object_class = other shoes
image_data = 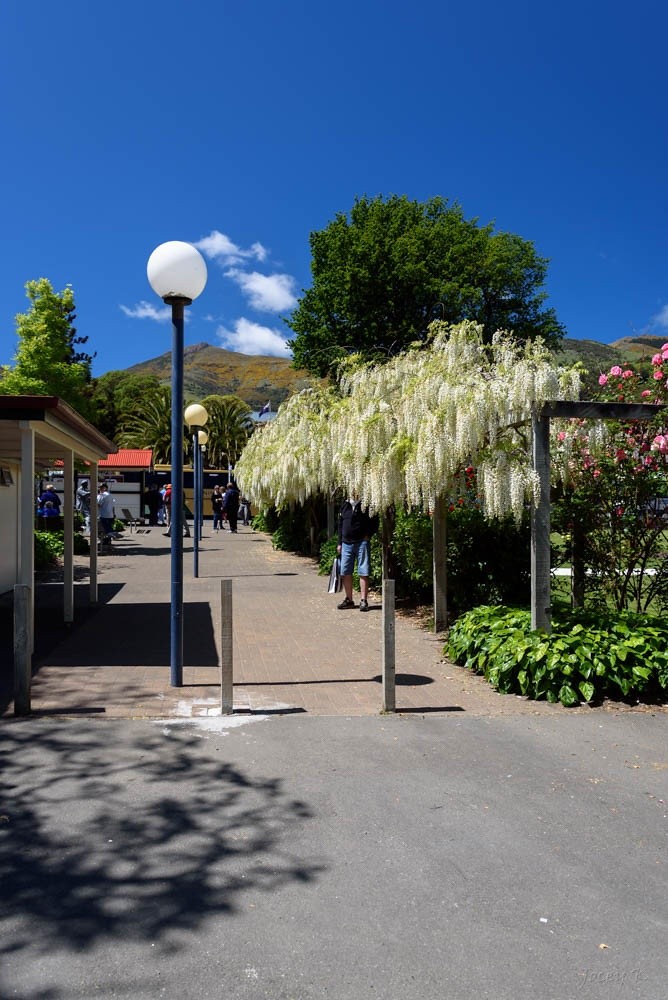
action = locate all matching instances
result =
[228,530,234,533]
[162,533,170,537]
[183,534,190,537]
[213,528,219,530]
[234,529,237,533]
[220,527,225,529]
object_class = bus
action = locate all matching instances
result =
[153,463,259,519]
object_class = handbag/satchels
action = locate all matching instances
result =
[327,555,343,594]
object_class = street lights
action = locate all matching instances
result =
[146,240,207,688]
[193,430,208,541]
[184,404,209,577]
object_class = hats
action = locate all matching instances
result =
[166,484,172,488]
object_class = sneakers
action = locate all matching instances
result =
[338,597,354,609]
[359,599,369,611]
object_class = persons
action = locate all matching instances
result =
[211,483,251,533]
[38,485,61,531]
[78,480,90,534]
[337,489,380,611]
[98,484,114,545]
[144,484,190,537]
